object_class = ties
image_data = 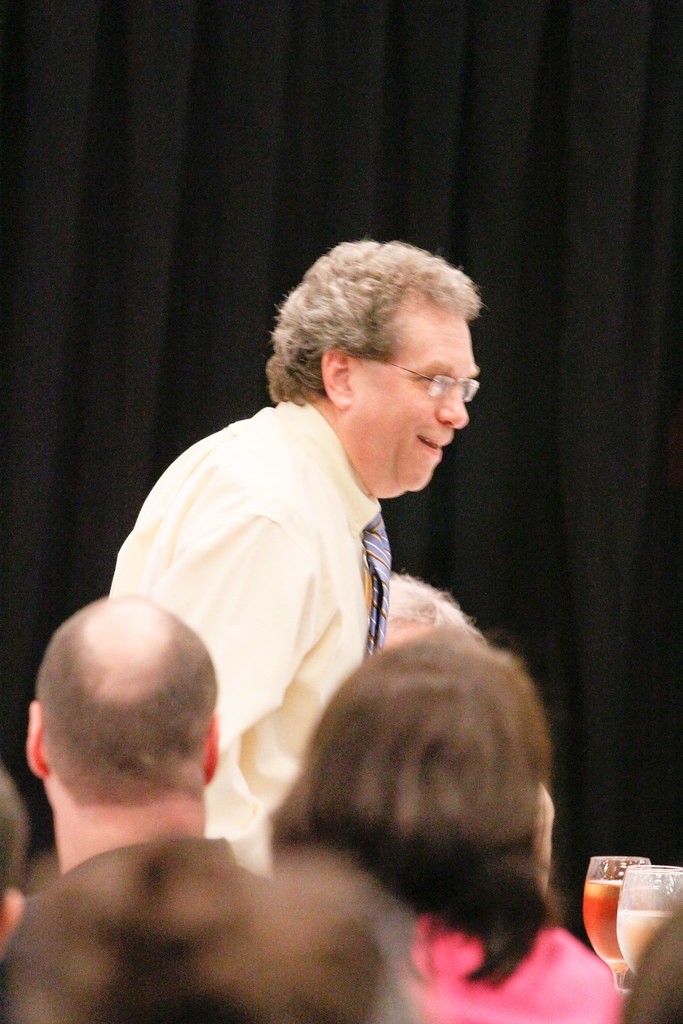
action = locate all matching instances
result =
[361,512,390,660]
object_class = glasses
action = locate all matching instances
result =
[367,352,480,402]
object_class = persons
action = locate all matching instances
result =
[0,575,683,1024]
[109,237,481,874]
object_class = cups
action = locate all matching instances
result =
[615,865,683,977]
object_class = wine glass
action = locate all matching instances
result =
[583,855,653,994]
